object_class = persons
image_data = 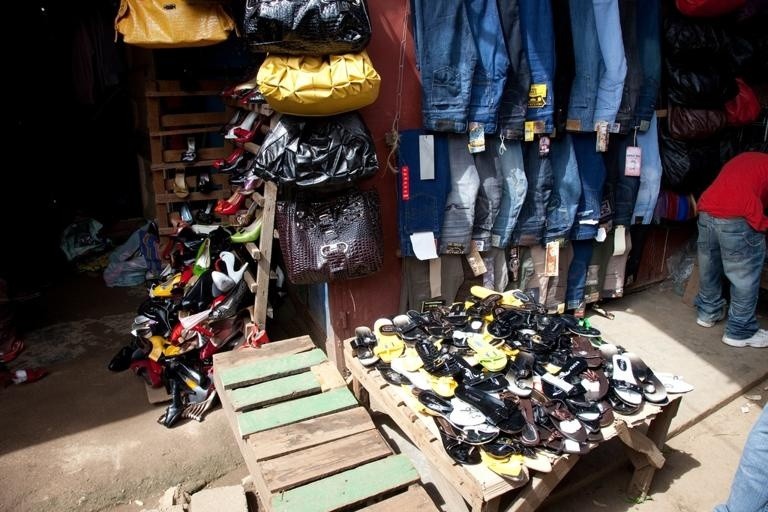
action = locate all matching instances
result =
[695,151,768,348]
[714,401,768,512]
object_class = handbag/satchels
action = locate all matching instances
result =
[273,190,388,286]
[240,0,376,57]
[250,110,383,193]
[254,48,383,120]
[112,0,243,52]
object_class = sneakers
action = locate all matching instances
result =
[720,328,768,348]
[695,303,726,328]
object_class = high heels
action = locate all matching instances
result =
[212,82,268,197]
[197,165,212,195]
[108,297,269,428]
[173,168,189,199]
[149,187,264,297]
[182,136,197,163]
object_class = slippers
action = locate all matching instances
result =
[422,286,556,482]
[615,345,693,416]
[350,309,421,383]
[558,313,615,456]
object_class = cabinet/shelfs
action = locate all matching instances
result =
[131,76,235,238]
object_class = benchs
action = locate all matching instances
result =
[209,333,440,510]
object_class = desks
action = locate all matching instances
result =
[341,289,681,511]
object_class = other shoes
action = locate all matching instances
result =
[178,70,200,95]
